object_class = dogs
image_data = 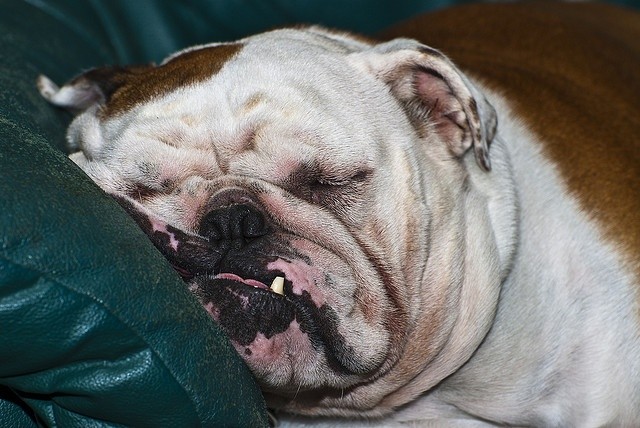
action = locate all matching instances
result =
[37,25,639,428]
